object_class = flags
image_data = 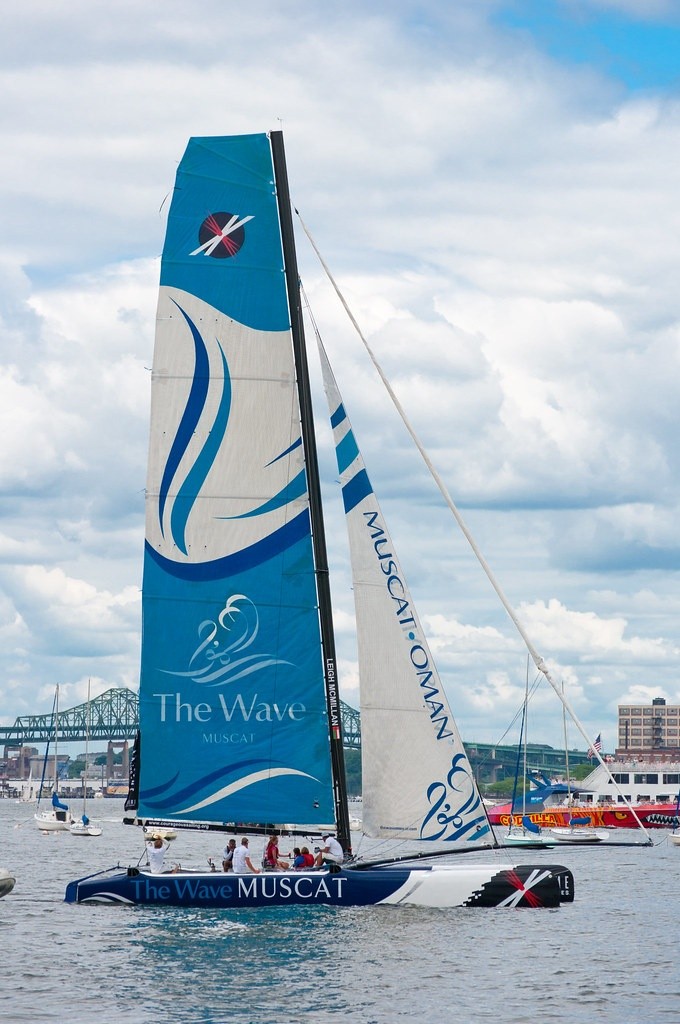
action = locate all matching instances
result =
[588,734,602,758]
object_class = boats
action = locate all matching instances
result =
[316,814,362,831]
[486,732,680,829]
[668,833,680,846]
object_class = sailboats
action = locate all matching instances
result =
[33,681,76,830]
[37,756,129,799]
[549,681,611,842]
[503,653,559,850]
[19,767,37,803]
[65,130,575,912]
[69,678,104,836]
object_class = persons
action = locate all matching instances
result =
[313,831,347,863]
[233,838,260,874]
[145,836,179,874]
[299,847,314,867]
[222,838,236,871]
[290,848,304,868]
[264,836,290,867]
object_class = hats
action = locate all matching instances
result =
[321,832,329,838]
